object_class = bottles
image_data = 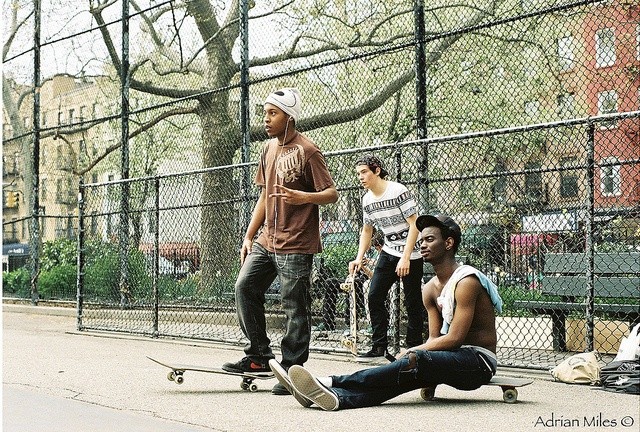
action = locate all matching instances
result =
[265,87,303,126]
[353,154,389,179]
[415,213,461,243]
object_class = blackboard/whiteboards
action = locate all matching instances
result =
[128,241,201,280]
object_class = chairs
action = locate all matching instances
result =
[221,356,275,377]
[287,364,341,412]
[365,345,387,357]
[271,381,293,394]
[268,358,315,408]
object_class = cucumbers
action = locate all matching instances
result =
[146,357,275,392]
[340,265,358,356]
[421,376,533,403]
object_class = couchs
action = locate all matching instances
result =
[5,192,19,208]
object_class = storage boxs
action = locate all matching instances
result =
[221,252,284,301]
[512,252,639,355]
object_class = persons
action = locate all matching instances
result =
[348,154,423,363]
[267,274,280,297]
[222,87,339,395]
[359,233,385,350]
[268,215,497,412]
[314,225,377,343]
[309,257,338,341]
